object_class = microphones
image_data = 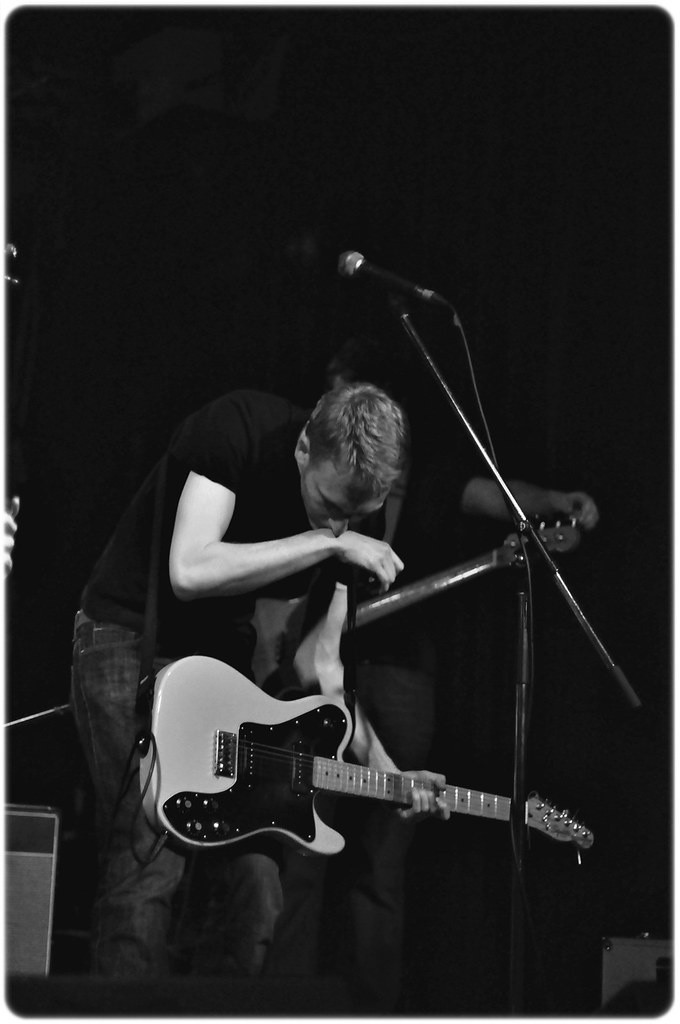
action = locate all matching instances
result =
[339,250,456,313]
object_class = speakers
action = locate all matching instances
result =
[6,804,61,976]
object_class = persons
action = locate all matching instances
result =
[59,379,406,986]
[4,492,25,582]
[268,285,600,1014]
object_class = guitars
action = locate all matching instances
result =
[133,655,596,868]
